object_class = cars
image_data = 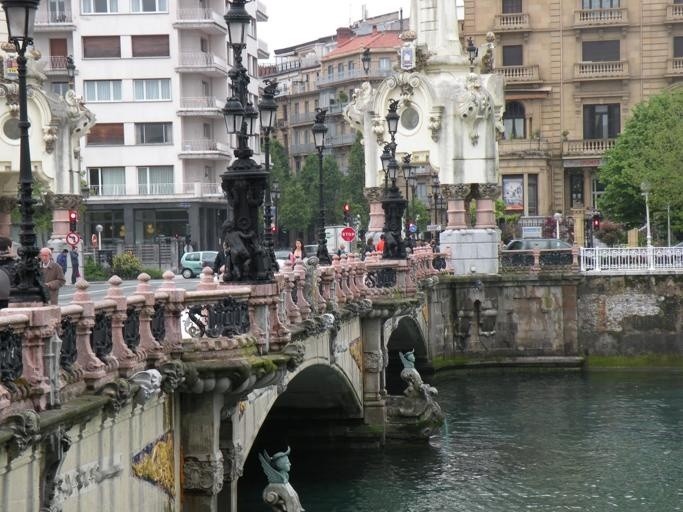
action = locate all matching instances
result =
[275,242,318,269]
[501,236,591,264]
[180,251,220,279]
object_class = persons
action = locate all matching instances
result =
[267,445,293,482]
[375,233,384,253]
[55,248,69,277]
[37,246,67,306]
[287,237,308,273]
[207,246,226,282]
[403,231,413,248]
[360,237,375,261]
[69,247,79,284]
[496,216,512,245]
[403,350,417,364]
[335,243,345,257]
[0,235,21,308]
[552,208,562,226]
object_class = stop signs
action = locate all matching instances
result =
[342,227,356,242]
[91,234,97,247]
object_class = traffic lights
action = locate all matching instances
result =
[67,208,79,231]
[343,202,351,222]
[594,213,599,229]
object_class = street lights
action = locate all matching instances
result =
[553,210,562,245]
[639,180,655,268]
[379,103,413,256]
[1,0,48,307]
[311,108,331,263]
[95,223,103,249]
[221,0,279,283]
[431,172,442,267]
